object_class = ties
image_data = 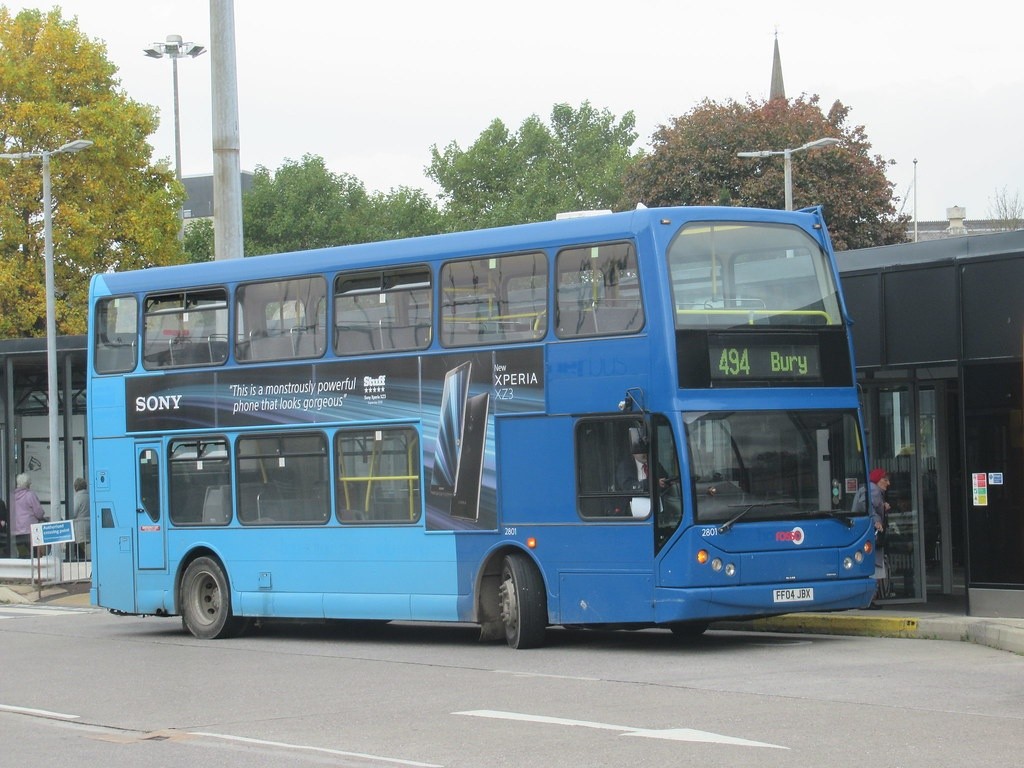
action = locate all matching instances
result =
[643,463,648,478]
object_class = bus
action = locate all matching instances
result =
[85,202,873,649]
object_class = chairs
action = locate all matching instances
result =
[131,298,771,366]
[254,489,408,521]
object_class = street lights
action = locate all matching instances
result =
[0,140,92,566]
[142,35,207,251]
[736,136,839,209]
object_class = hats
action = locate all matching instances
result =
[632,443,649,454]
[870,469,886,483]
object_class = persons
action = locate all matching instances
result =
[0,499,8,533]
[13,472,45,558]
[69,477,90,559]
[849,467,895,612]
[615,434,673,516]
